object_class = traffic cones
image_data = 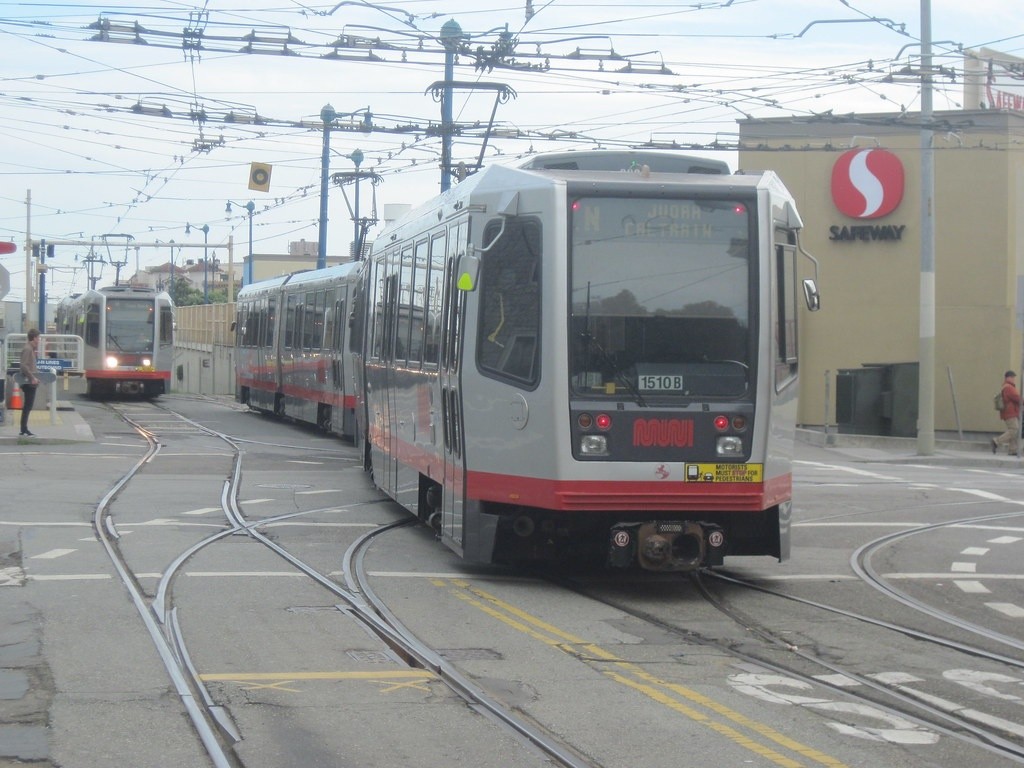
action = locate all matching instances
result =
[7,381,23,410]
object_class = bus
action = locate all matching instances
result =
[231,259,363,441]
[53,292,82,360]
[355,148,821,576]
[65,286,174,401]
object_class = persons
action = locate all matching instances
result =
[990,371,1021,456]
[17,330,40,435]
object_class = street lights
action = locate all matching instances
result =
[155,238,175,306]
[185,221,209,304]
[225,200,256,285]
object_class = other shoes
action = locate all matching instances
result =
[1009,453,1018,455]
[991,440,997,453]
[20,431,35,437]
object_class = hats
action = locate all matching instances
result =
[1005,372,1016,376]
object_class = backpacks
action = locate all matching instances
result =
[994,384,1012,410]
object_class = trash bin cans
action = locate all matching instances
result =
[836,368,885,436]
[863,362,921,438]
